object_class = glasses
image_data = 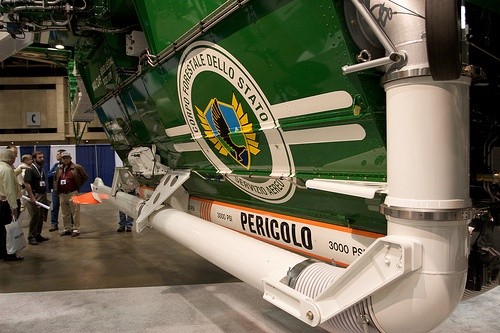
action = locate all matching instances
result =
[38,157,45,159]
[62,157,69,159]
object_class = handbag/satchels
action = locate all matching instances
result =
[42,198,51,222]
[5,214,29,255]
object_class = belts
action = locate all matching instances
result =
[62,189,76,194]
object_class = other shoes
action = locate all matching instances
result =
[4,254,24,261]
[59,231,71,236]
[126,227,132,232]
[72,232,80,237]
[37,236,49,241]
[48,226,58,231]
[117,227,125,232]
[28,239,39,245]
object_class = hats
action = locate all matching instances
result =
[61,151,72,159]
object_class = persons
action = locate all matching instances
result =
[0,145,49,261]
[54,152,88,237]
[117,189,138,232]
[47,149,74,231]
